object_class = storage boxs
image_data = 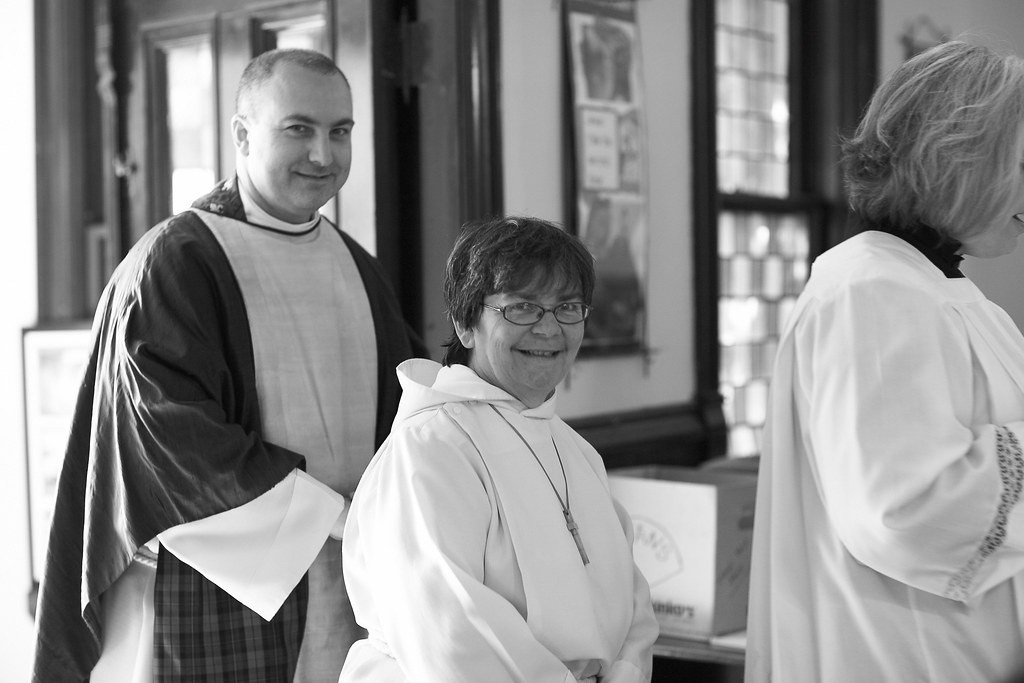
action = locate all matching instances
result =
[605,465,761,641]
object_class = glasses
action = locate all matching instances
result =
[484,302,593,326]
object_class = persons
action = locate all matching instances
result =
[338,215,660,683]
[32,49,435,683]
[744,35,1024,683]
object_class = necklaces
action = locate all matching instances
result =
[488,404,593,565]
